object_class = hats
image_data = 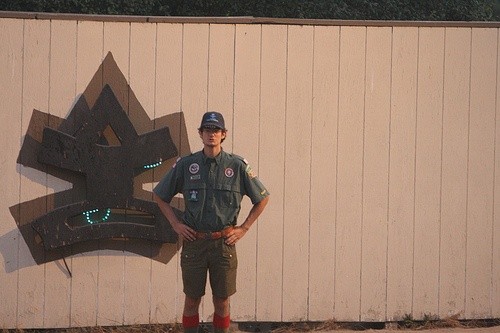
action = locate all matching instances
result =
[200,111,225,130]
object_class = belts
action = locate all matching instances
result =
[191,225,234,239]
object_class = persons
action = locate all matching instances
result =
[152,111,270,333]
